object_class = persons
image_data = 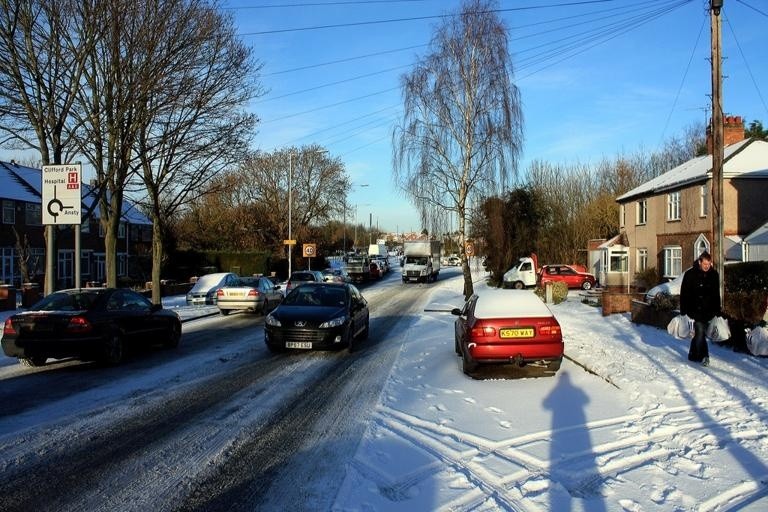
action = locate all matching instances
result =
[679,251,722,367]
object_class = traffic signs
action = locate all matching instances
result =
[303,243,316,257]
[464,241,476,256]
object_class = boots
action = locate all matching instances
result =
[703,357,709,366]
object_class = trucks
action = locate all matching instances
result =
[502,252,588,289]
[402,241,440,283]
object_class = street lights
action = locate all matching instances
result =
[343,183,370,255]
[289,150,330,279]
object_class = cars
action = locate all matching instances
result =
[451,290,563,370]
[442,257,462,266]
[1,287,182,367]
[644,259,743,309]
[540,263,595,290]
[186,257,390,351]
